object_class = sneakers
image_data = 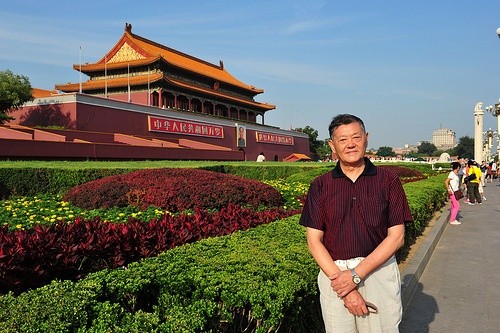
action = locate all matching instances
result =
[450,220,461,224]
[483,197,486,200]
[480,203,482,204]
[463,199,470,203]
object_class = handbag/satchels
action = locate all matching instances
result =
[464,174,476,183]
[454,190,464,200]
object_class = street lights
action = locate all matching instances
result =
[487,98,500,160]
[482,128,496,161]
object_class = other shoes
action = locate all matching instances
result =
[469,203,475,206]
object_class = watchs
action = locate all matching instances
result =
[352,269,362,285]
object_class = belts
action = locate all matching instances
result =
[335,260,359,269]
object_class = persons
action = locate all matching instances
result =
[299,114,415,333]
[485,161,500,183]
[304,156,338,162]
[369,155,415,162]
[457,157,464,191]
[238,127,245,147]
[445,162,461,224]
[467,163,482,205]
[274,155,278,161]
[256,152,266,162]
[461,157,487,204]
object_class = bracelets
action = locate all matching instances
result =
[448,189,451,192]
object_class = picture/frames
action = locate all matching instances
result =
[237,124,247,148]
